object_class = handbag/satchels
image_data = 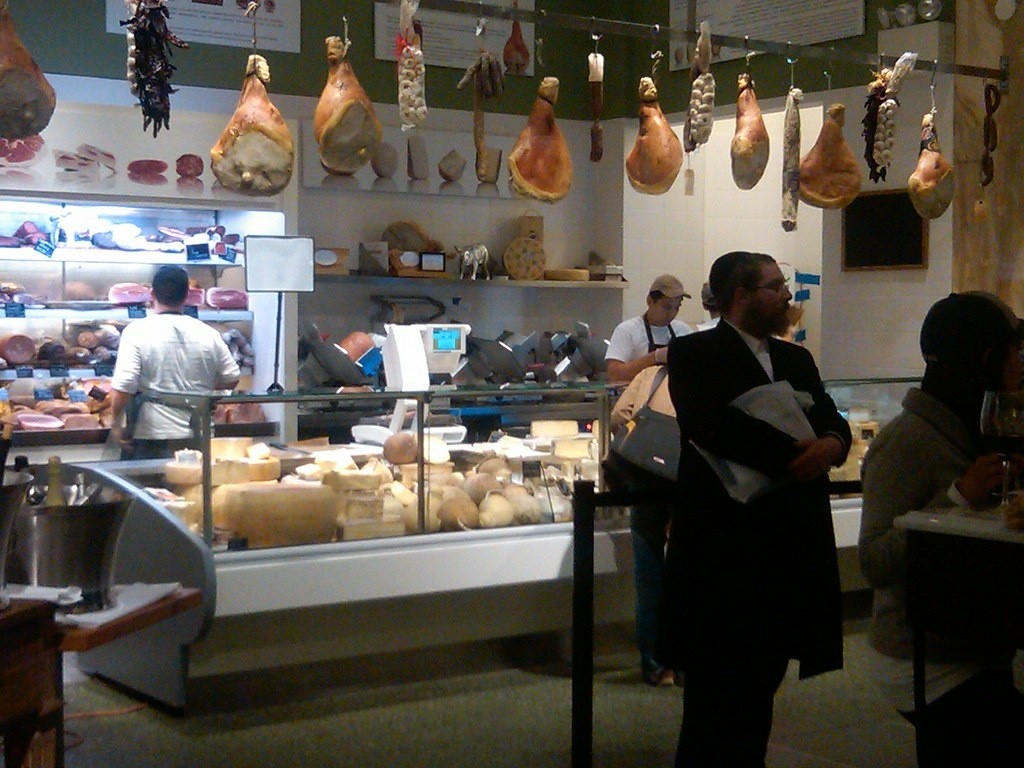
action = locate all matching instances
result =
[687,380,817,502]
[610,364,680,479]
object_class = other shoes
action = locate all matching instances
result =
[649,668,675,685]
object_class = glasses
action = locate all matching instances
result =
[752,279,789,291]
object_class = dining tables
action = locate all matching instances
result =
[892,503,1023,768]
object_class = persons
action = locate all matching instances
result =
[861,290,1024,768]
[604,274,697,384]
[693,282,721,331]
[601,359,683,684]
[107,265,240,462]
[671,252,853,768]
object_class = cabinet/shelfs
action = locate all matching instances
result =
[297,273,626,442]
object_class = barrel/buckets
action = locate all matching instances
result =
[0,469,135,613]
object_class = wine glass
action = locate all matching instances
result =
[980,390,1024,522]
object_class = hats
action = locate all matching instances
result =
[921,290,1024,369]
[650,275,691,298]
